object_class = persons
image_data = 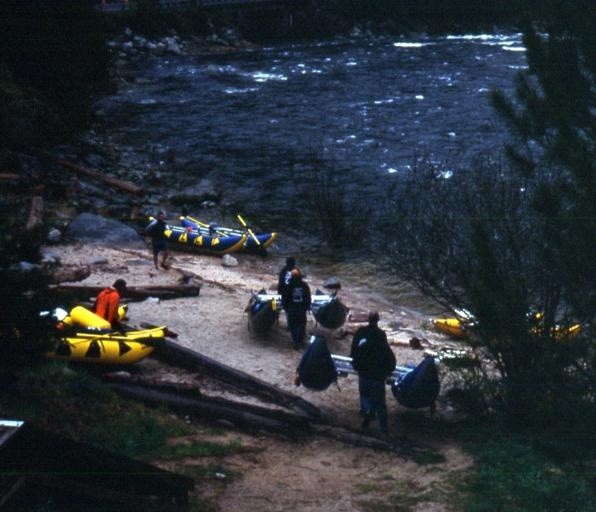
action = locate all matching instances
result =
[349,310,389,422]
[140,209,173,271]
[276,255,302,330]
[354,325,397,432]
[280,268,311,351]
[89,278,128,337]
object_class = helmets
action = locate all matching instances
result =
[368,310,380,322]
[291,267,300,276]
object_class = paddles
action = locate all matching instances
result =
[237,215,268,258]
[77,333,166,347]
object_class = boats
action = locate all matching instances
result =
[431,306,583,344]
[146,214,277,255]
[14,325,165,364]
[244,287,441,410]
